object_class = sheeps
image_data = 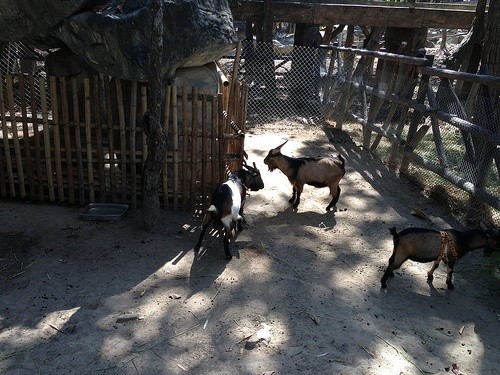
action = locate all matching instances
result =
[194,162,264,262]
[264,141,345,211]
[381,221,500,290]
